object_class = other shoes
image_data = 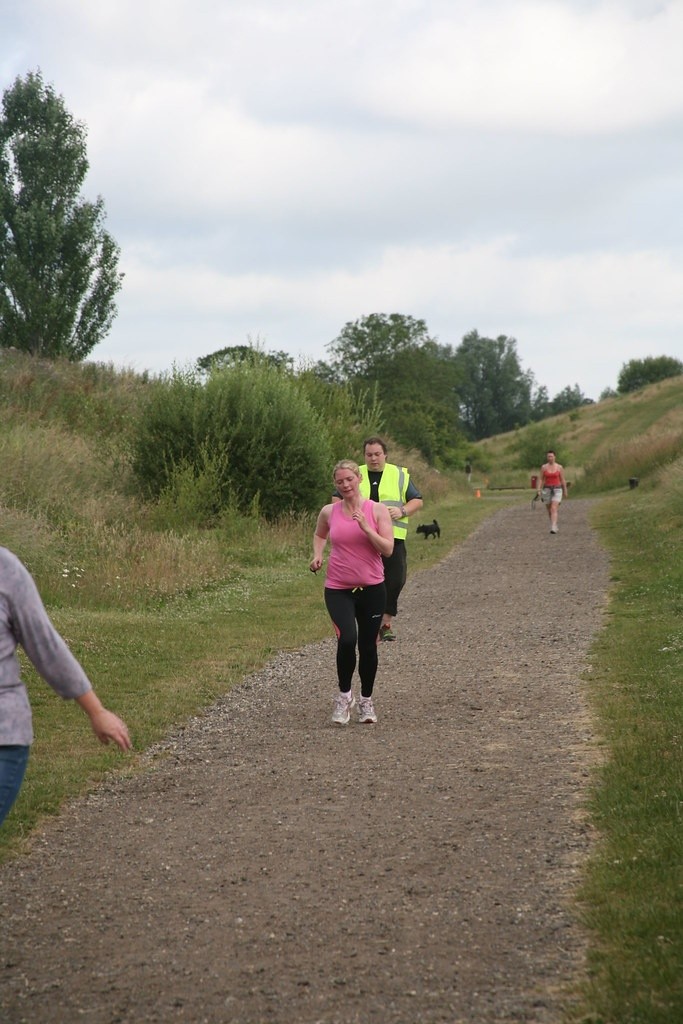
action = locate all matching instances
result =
[550,527,559,534]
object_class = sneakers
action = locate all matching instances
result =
[332,692,355,724]
[357,699,377,723]
[379,624,398,641]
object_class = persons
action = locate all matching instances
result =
[466,461,472,481]
[332,438,424,641]
[310,460,394,724]
[0,545,131,825]
[537,450,568,533]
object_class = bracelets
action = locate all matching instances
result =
[399,507,407,517]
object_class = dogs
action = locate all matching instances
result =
[416,519,441,540]
